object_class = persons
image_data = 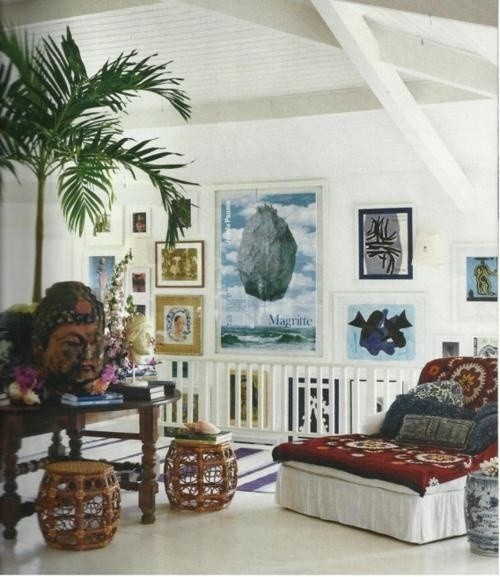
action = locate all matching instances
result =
[122,314,155,365]
[171,314,189,340]
[30,281,108,385]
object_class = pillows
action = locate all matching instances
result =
[395,414,476,450]
[468,403,498,454]
[379,393,472,450]
[408,380,465,408]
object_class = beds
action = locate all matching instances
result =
[272,356,498,545]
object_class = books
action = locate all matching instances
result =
[115,384,165,402]
[60,389,124,408]
[173,431,233,446]
[1,392,11,408]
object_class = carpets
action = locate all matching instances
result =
[63,436,278,495]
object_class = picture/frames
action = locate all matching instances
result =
[451,243,499,323]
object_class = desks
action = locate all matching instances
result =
[0,387,181,539]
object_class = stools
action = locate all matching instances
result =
[464,472,499,557]
[163,440,239,512]
[36,460,123,550]
